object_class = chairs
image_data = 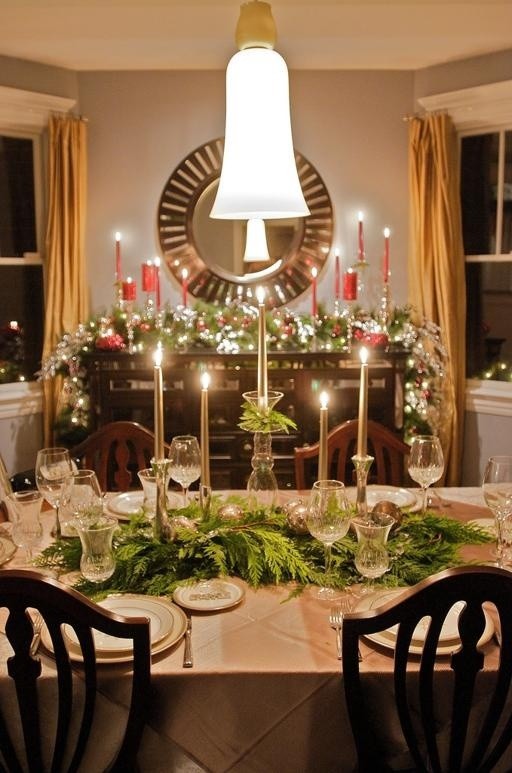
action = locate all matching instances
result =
[0,569,151,772]
[294,418,433,490]
[341,565,512,773]
[69,421,187,491]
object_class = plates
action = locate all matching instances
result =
[482,456,512,559]
[0,537,16,566]
[402,495,423,515]
[383,601,470,641]
[63,598,174,653]
[347,484,417,508]
[174,578,245,610]
[109,491,182,515]
[352,589,495,656]
[467,518,512,542]
[40,592,188,664]
[422,654,423,655]
[103,496,189,520]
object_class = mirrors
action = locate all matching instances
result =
[156,133,337,312]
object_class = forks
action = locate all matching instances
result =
[29,614,45,655]
[329,606,342,659]
[182,612,193,667]
[340,599,363,662]
[426,489,436,508]
[432,488,451,506]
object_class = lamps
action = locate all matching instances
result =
[208,0,312,263]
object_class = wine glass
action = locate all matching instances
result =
[168,436,202,506]
[80,518,118,591]
[349,511,395,596]
[307,479,352,587]
[137,468,170,512]
[408,436,444,511]
[3,491,45,560]
[35,448,73,508]
[59,470,104,528]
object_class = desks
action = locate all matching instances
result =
[81,344,413,490]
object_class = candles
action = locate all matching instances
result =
[116,241,122,281]
[200,389,210,487]
[182,278,187,308]
[343,272,357,300]
[154,367,165,464]
[359,221,364,261]
[141,263,154,292]
[155,266,160,311]
[357,364,369,459]
[311,277,317,316]
[257,304,268,398]
[122,282,137,301]
[318,408,327,511]
[384,238,390,283]
[335,256,339,299]
